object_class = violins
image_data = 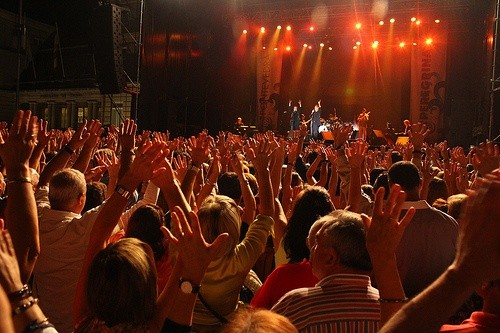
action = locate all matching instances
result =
[363,113,369,121]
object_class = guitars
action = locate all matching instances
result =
[299,118,312,128]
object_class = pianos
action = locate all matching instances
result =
[236,126,258,134]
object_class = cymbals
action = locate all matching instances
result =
[329,117,338,120]
[320,118,325,122]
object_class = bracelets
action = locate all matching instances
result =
[240,180,249,185]
[38,142,46,148]
[206,179,217,187]
[443,158,449,163]
[62,143,75,155]
[12,284,28,297]
[412,149,423,154]
[6,178,32,183]
[188,164,200,173]
[120,150,135,156]
[29,324,55,333]
[13,298,40,316]
[287,163,295,167]
[11,285,33,301]
[377,298,408,303]
[28,317,49,326]
[299,135,305,139]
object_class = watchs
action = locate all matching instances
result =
[114,183,133,201]
[179,279,201,294]
[336,145,346,149]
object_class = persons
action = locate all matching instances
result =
[0,98,500,333]
